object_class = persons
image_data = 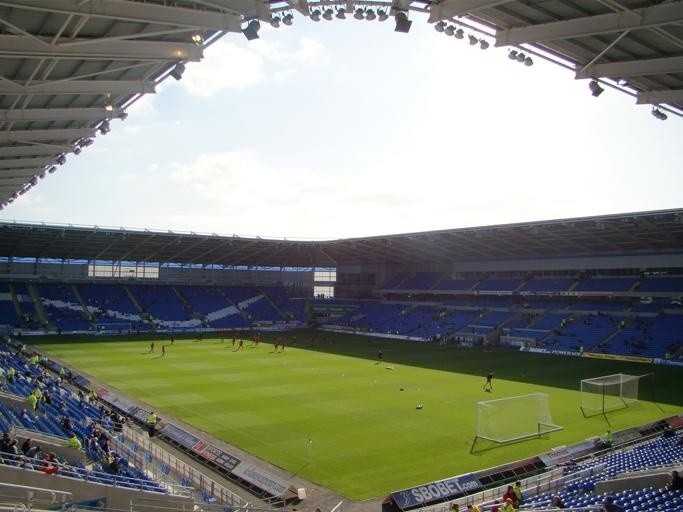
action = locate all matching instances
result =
[0,336,157,467]
[453,425,683,512]
[316,292,683,360]
[21,284,174,357]
[180,295,296,352]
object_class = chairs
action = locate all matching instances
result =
[330,267,683,362]
[0,341,172,494]
[1,276,306,335]
[472,424,683,511]
